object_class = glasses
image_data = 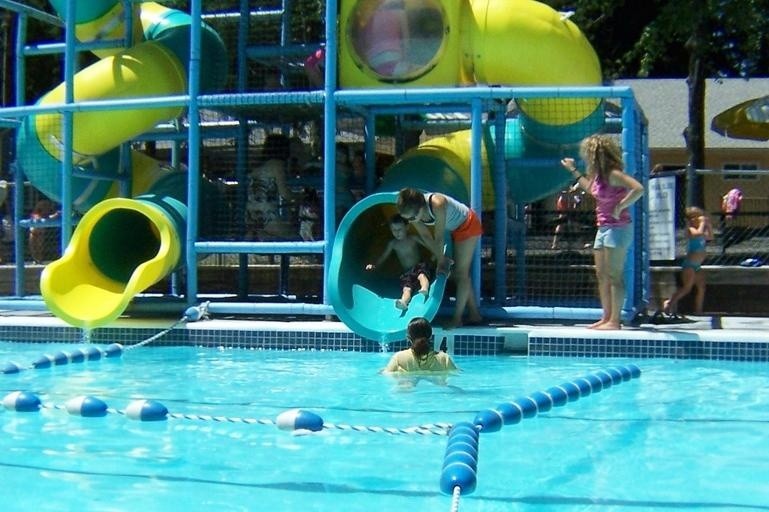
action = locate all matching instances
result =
[401,216,416,221]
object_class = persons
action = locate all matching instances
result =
[30,200,64,262]
[551,188,571,248]
[352,156,366,198]
[366,214,453,311]
[245,132,295,238]
[334,145,354,222]
[663,206,715,320]
[355,1,423,75]
[561,132,645,330]
[397,188,491,329]
[217,156,226,177]
[298,195,318,241]
[304,21,323,88]
[379,317,464,386]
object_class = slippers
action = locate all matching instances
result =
[633,310,690,325]
